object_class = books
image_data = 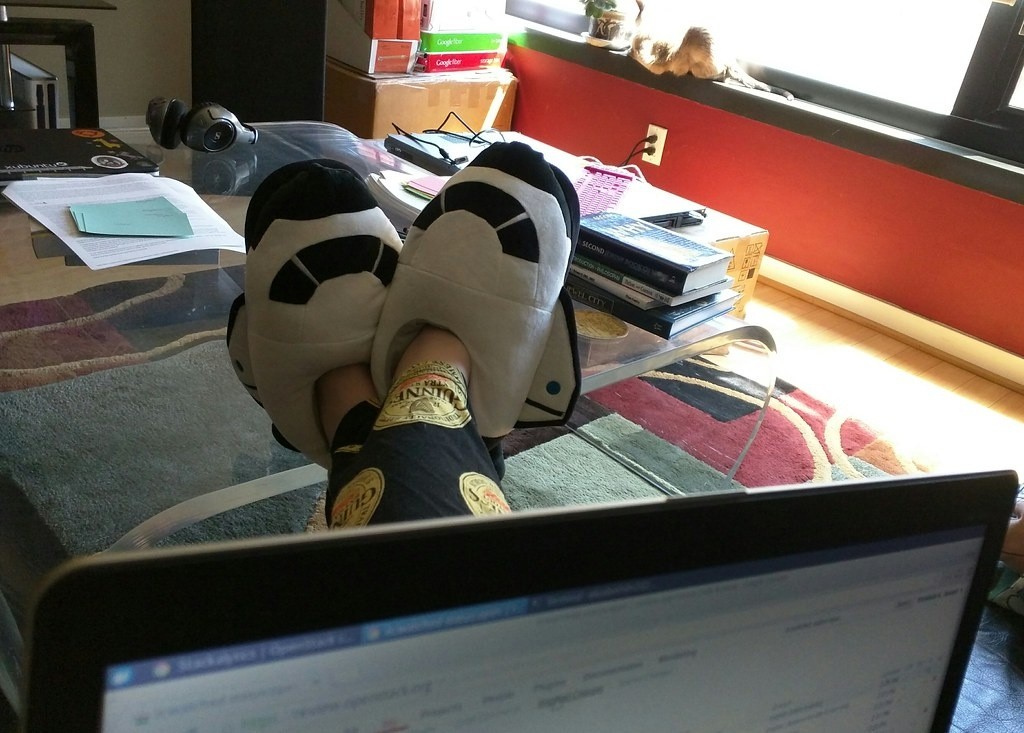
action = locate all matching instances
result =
[562,211,743,341]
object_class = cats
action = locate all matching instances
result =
[623,0,795,102]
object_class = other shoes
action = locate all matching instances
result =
[230,158,402,473]
[373,141,579,460]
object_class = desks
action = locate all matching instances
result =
[0,123,1023,733]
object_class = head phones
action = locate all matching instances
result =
[146,97,258,153]
[191,149,258,196]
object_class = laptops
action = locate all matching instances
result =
[21,471,1019,733]
[384,131,496,176]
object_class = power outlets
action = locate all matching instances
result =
[642,124,668,165]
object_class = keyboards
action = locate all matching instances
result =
[572,167,634,217]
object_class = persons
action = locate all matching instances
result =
[227,140,583,534]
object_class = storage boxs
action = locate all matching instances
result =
[618,181,769,317]
[101,116,194,188]
[323,0,519,139]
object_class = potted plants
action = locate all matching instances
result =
[579,0,626,41]
